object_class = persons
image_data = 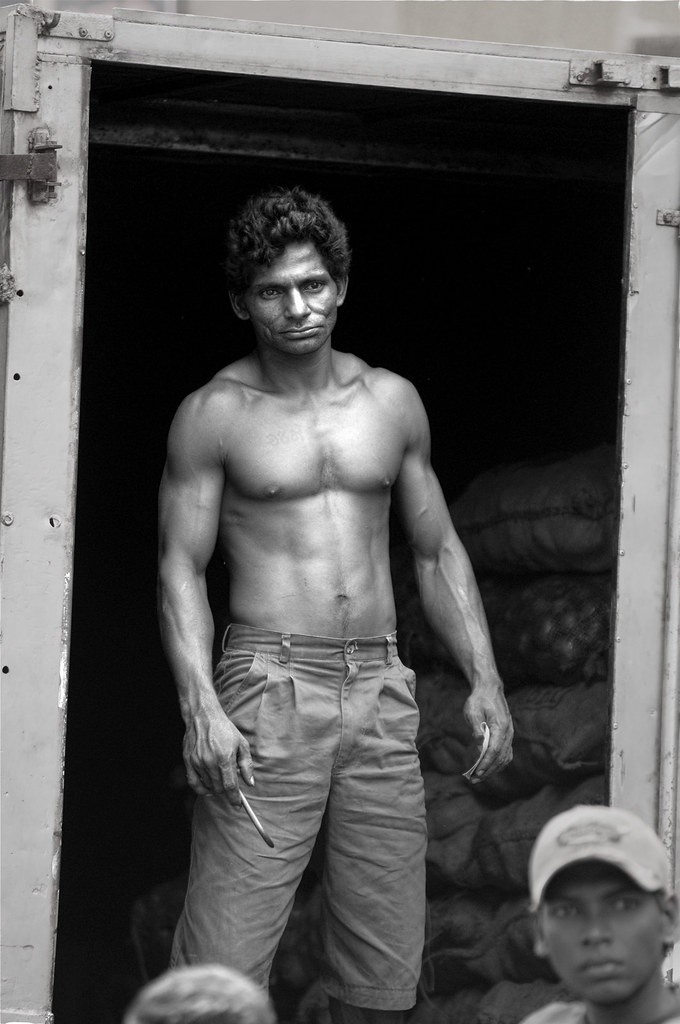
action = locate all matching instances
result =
[157,189,512,1024]
[125,964,277,1024]
[519,805,680,1024]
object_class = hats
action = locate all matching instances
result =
[529,804,671,914]
